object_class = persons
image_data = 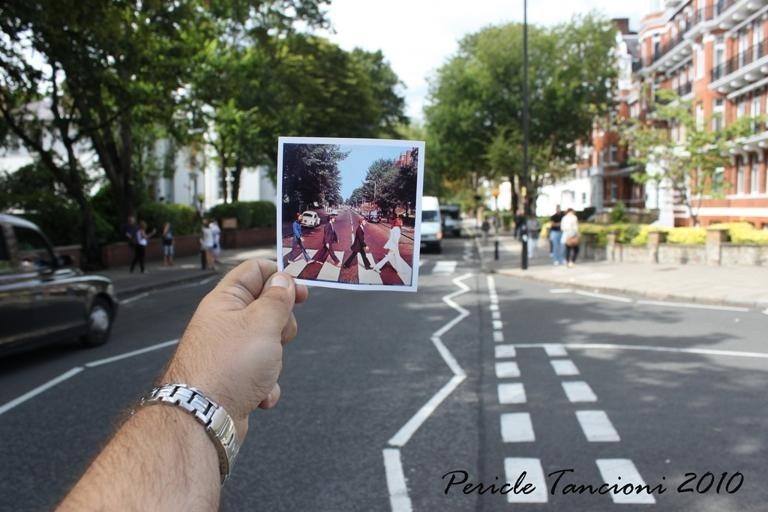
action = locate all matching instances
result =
[52,258,309,512]
[481,204,579,267]
[374,218,402,275]
[288,213,314,263]
[344,218,374,270]
[316,215,342,266]
[125,214,223,272]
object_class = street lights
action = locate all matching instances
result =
[363,180,376,205]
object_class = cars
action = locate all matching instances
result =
[1,215,119,357]
[299,211,321,228]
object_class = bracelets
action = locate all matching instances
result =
[130,383,242,486]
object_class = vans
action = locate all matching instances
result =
[368,210,381,223]
[421,196,462,252]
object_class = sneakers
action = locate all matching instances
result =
[336,262,350,268]
[365,265,382,273]
[307,260,324,265]
[288,259,295,264]
